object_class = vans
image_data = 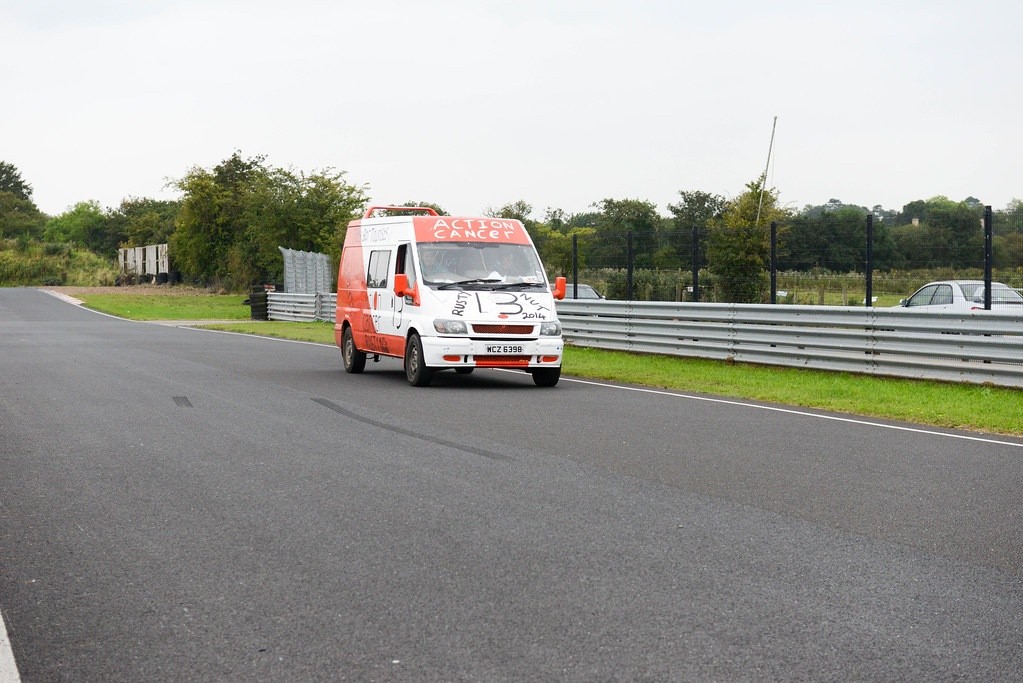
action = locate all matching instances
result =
[333,206,566,390]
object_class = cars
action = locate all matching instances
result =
[896,280,1023,309]
[548,282,605,301]
